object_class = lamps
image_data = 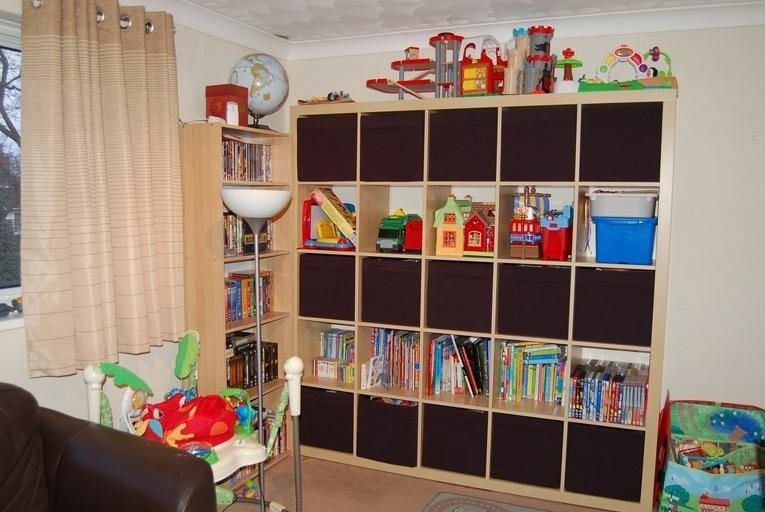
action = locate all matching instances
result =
[218,189,297,511]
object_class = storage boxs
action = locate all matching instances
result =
[586,187,659,267]
[658,397,765,510]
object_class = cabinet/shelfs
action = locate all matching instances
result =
[293,96,675,511]
[181,126,294,486]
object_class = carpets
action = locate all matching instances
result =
[422,489,551,512]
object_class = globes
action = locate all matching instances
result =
[229,53,289,130]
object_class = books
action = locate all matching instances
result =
[314,329,354,384]
[86,335,253,512]
[425,332,491,398]
[569,365,647,427]
[360,327,420,390]
[223,141,288,484]
[496,339,568,406]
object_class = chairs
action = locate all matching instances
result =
[0,374,220,510]
